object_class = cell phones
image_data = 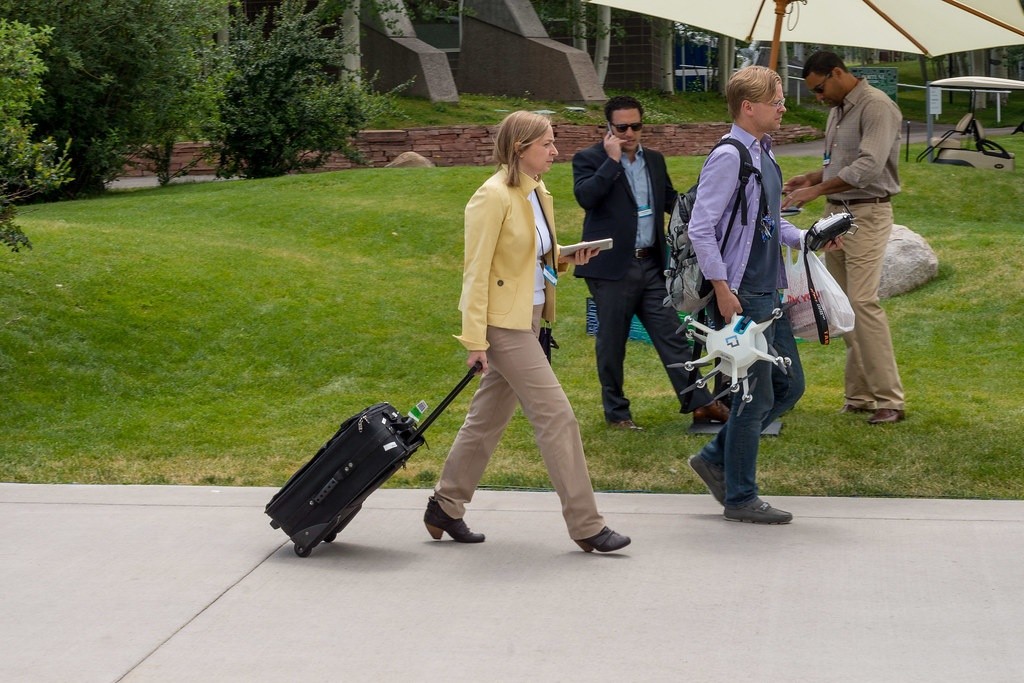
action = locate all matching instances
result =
[606,123,613,137]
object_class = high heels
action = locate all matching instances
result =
[423,493,486,544]
[574,528,630,552]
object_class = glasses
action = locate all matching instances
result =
[744,96,787,109]
[808,68,834,95]
[608,121,644,132]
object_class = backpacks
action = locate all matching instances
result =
[662,134,761,314]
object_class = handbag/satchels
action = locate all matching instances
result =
[782,230,855,342]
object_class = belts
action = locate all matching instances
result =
[825,195,891,206]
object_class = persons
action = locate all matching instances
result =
[572,95,728,427]
[423,111,631,552]
[690,68,843,525]
[781,50,906,424]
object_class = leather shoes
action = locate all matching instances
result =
[612,418,643,430]
[841,404,874,415]
[867,406,905,424]
[693,400,733,426]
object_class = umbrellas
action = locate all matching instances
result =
[588,0,1024,72]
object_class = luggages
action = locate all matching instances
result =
[264,361,483,558]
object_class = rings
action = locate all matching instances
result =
[789,196,792,199]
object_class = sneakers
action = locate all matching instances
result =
[687,454,729,507]
[722,495,794,525]
